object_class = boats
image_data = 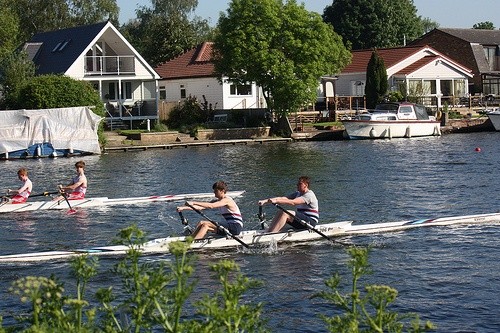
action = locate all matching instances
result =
[342,101,442,138]
[0,220,353,264]
[1,112,99,158]
[487,110,500,133]
[0,190,245,214]
[331,214,500,243]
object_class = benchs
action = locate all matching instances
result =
[209,114,227,129]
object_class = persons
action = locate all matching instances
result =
[177,181,244,240]
[52,161,87,201]
[258,175,319,234]
[0,169,32,203]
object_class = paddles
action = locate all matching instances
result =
[27,191,59,198]
[60,192,77,214]
[273,203,354,246]
[189,205,253,249]
[258,205,264,229]
[177,209,193,235]
